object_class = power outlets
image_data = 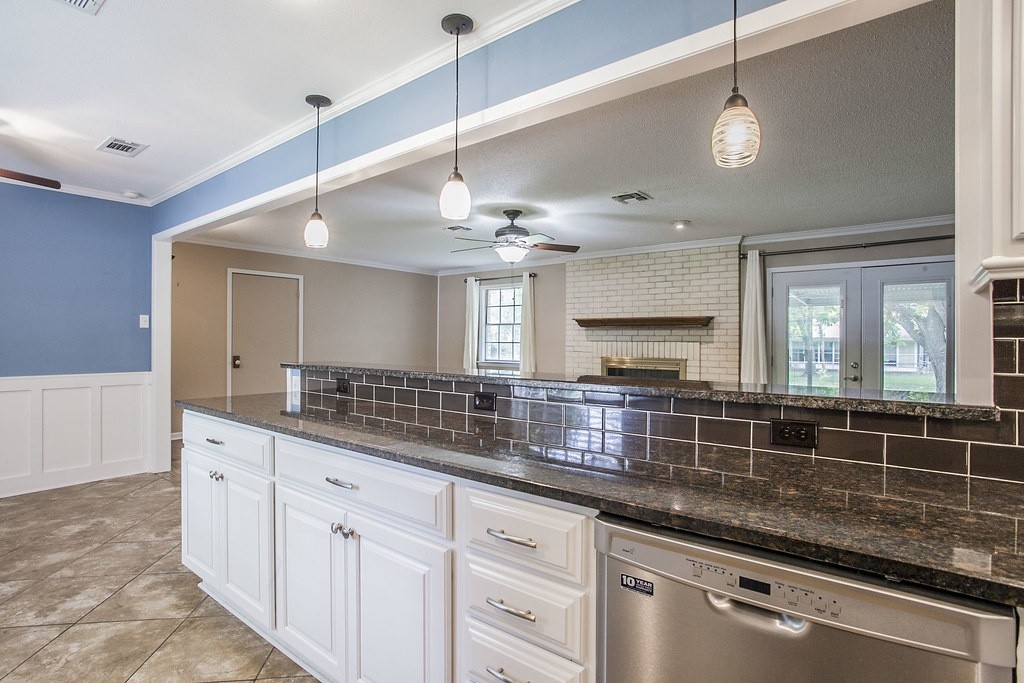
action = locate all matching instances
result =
[474,390,496,411]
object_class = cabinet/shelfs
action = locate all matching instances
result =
[181,408,597,683]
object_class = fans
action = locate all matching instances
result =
[450,209,580,253]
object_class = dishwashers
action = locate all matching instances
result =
[593,513,1017,683]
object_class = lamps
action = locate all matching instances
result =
[494,210,530,265]
[304,95,330,247]
[711,1,760,167]
[441,14,474,219]
